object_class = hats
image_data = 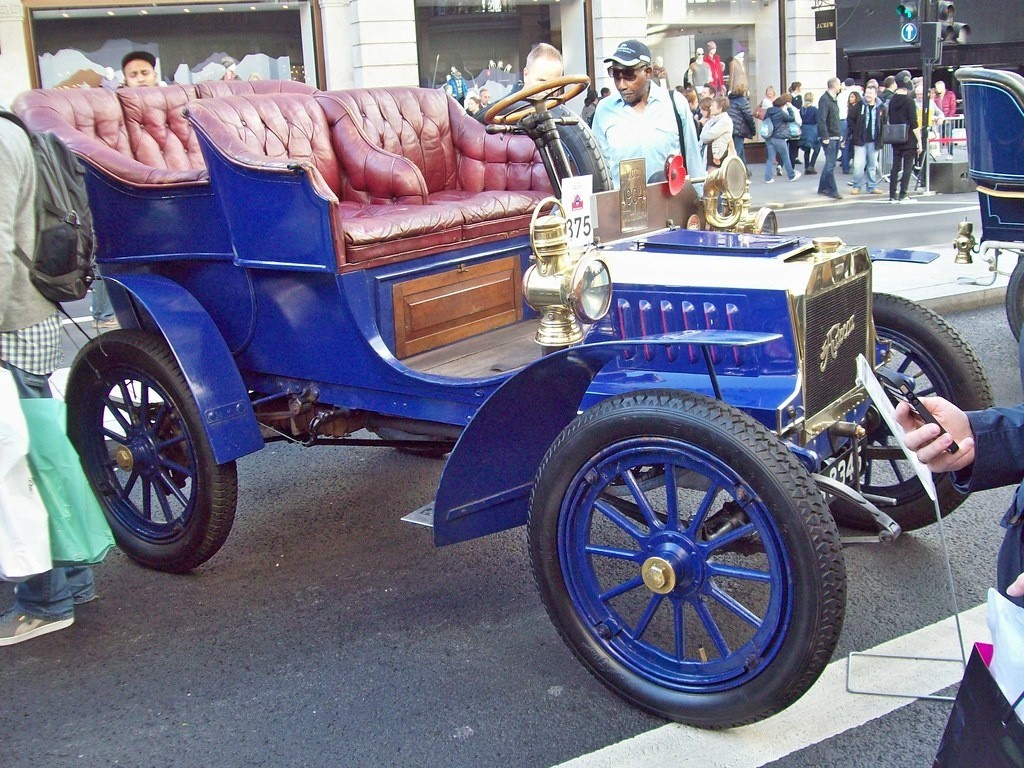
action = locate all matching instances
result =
[845,78,855,86]
[895,70,911,82]
[735,44,744,56]
[707,41,717,51]
[604,40,651,66]
[121,50,156,76]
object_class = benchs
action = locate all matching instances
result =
[10,77,573,275]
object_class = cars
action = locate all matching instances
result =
[11,75,999,729]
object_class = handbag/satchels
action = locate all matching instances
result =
[753,102,767,120]
[0,368,54,582]
[20,398,117,566]
[787,122,802,137]
[882,124,909,144]
[933,642,1024,768]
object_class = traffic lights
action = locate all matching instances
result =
[896,0,921,44]
[937,2,972,46]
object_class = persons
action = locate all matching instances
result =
[444,85,490,116]
[520,43,564,100]
[817,76,844,200]
[688,48,713,86]
[889,70,918,203]
[728,44,750,97]
[701,41,726,95]
[115,51,164,88]
[581,89,598,123]
[597,87,610,101]
[671,76,956,200]
[891,316,1024,607]
[591,39,704,197]
[651,55,670,90]
[1,107,96,647]
[684,57,697,92]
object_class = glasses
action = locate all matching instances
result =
[607,65,645,79]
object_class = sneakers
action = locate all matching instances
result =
[0,610,74,647]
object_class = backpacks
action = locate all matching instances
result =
[0,105,97,301]
[760,111,783,139]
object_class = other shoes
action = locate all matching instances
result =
[871,189,884,193]
[851,188,859,194]
[817,191,843,199]
[765,159,817,184]
[888,196,916,205]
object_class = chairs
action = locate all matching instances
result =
[954,68,1024,183]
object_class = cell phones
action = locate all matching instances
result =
[876,366,959,454]
